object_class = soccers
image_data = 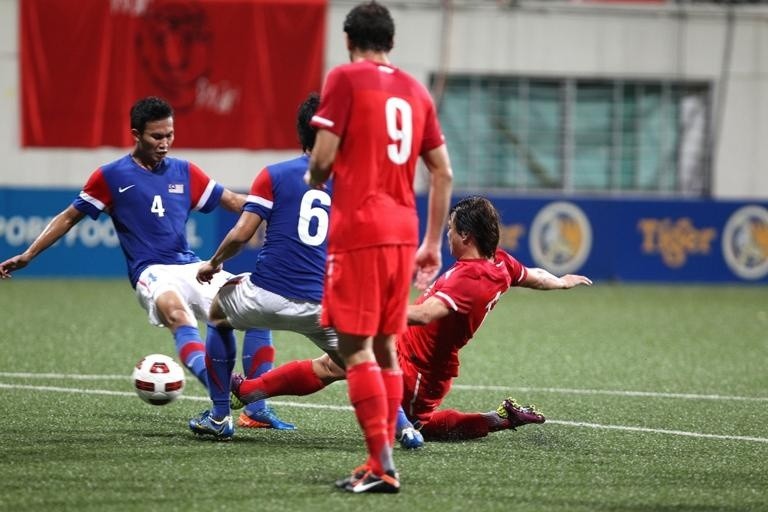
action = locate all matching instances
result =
[132,354,185,405]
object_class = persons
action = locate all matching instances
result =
[187,89,426,450]
[296,2,456,494]
[229,193,594,444]
[1,95,296,432]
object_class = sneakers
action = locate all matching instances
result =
[227,372,247,411]
[398,427,425,449]
[344,467,401,494]
[188,411,235,442]
[496,396,547,431]
[237,407,298,431]
[335,465,368,489]
